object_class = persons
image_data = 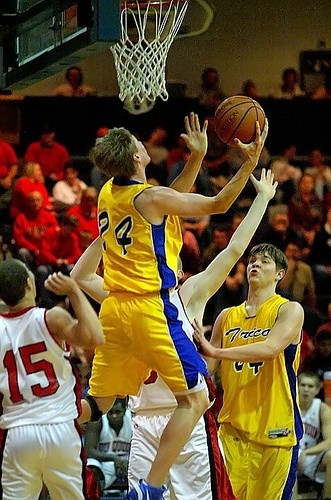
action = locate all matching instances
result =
[85,399,133,500]
[0,66,331,369]
[298,372,331,500]
[0,258,104,500]
[73,112,269,500]
[191,243,304,500]
[70,168,278,500]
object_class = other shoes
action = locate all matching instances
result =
[126,479,169,500]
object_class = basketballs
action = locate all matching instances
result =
[214,95,266,148]
